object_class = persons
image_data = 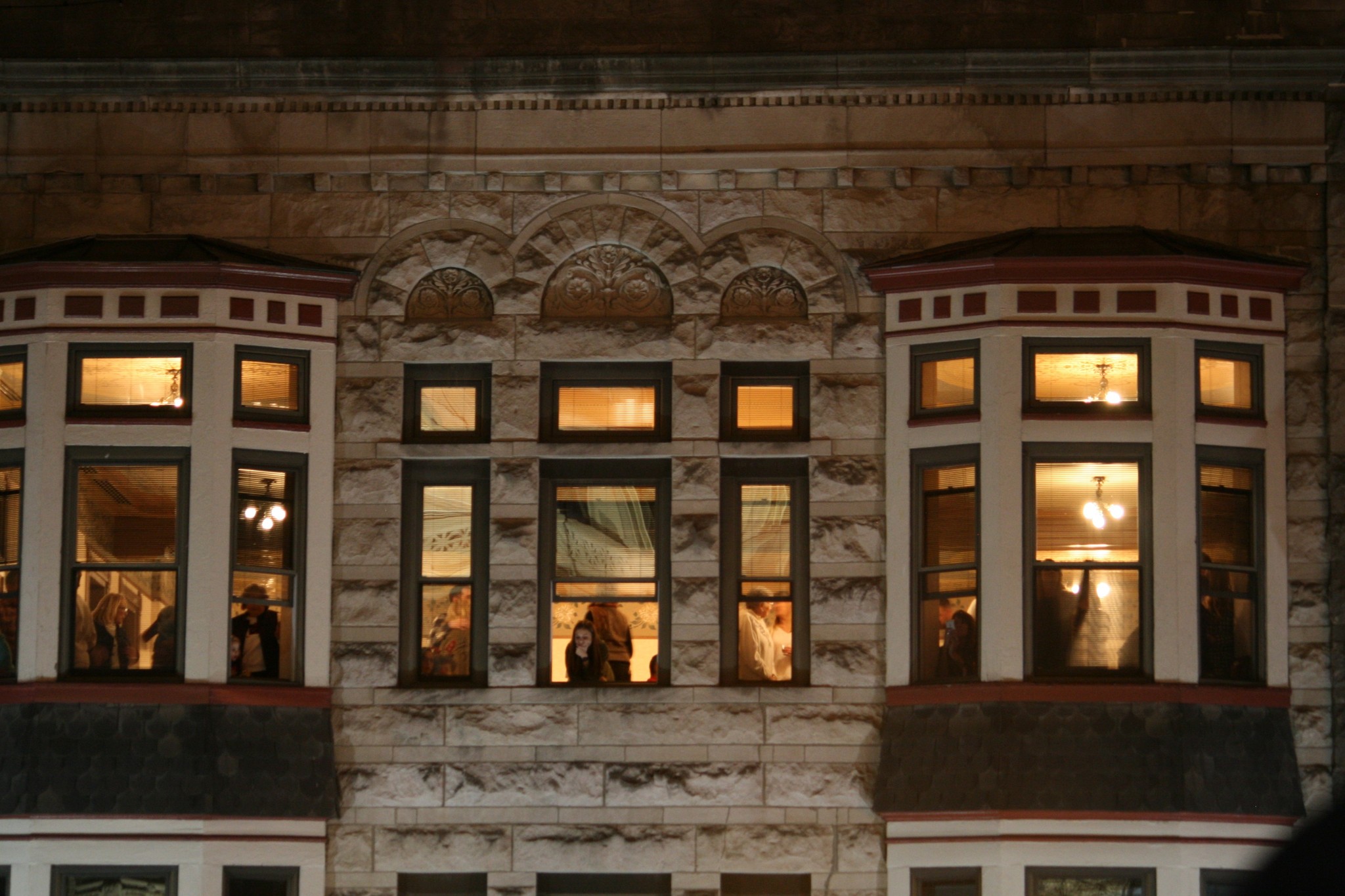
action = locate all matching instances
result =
[739,587,793,681]
[231,584,279,682]
[565,601,659,685]
[936,548,1236,682]
[1,565,19,685]
[428,585,471,674]
[72,561,178,671]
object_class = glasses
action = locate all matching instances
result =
[119,607,128,612]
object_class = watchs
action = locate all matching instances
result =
[582,656,589,662]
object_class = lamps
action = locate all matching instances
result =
[244,477,287,530]
[1082,475,1125,528]
[149,369,183,407]
[1084,364,1121,402]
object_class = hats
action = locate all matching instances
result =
[241,584,269,609]
